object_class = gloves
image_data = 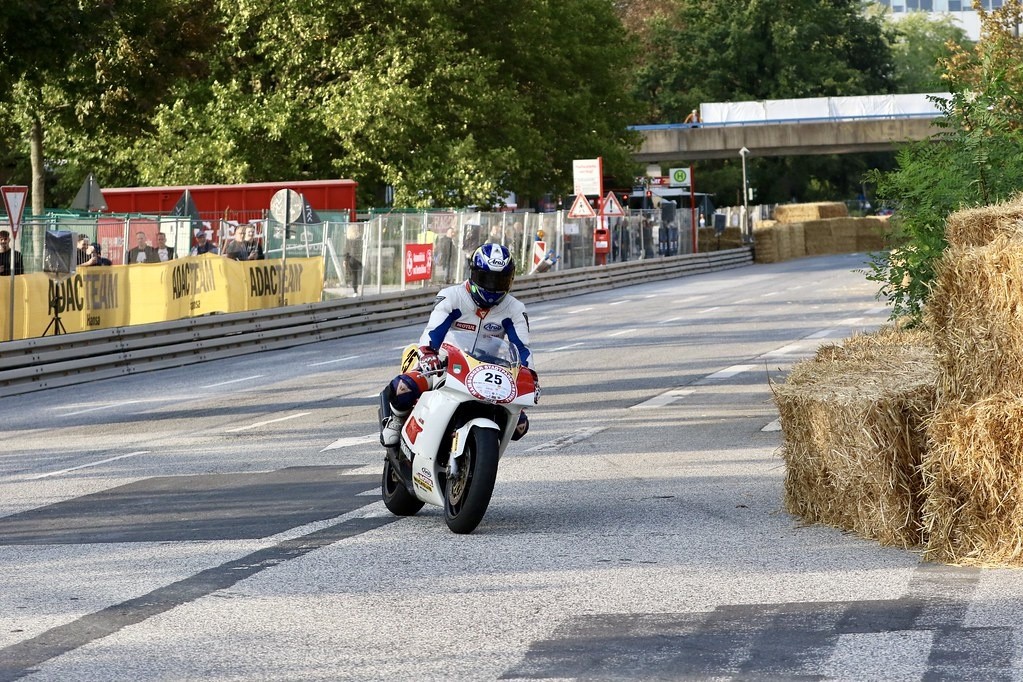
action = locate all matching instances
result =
[418,347,442,377]
[533,380,540,404]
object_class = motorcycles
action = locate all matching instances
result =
[375,332,545,536]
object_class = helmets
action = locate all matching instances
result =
[469,244,516,306]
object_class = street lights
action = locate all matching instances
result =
[737,147,751,246]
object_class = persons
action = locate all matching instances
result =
[76,231,178,266]
[227,225,265,262]
[188,231,221,256]
[684,110,702,127]
[343,229,364,297]
[609,216,680,263]
[418,218,570,281]
[380,243,541,447]
[698,214,707,228]
[0,230,24,276]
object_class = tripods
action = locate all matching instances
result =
[42,272,67,336]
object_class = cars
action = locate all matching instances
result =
[469,190,518,213]
[534,191,559,213]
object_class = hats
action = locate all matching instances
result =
[196,231,206,238]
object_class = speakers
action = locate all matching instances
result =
[44,230,74,273]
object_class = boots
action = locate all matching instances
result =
[382,402,410,445]
